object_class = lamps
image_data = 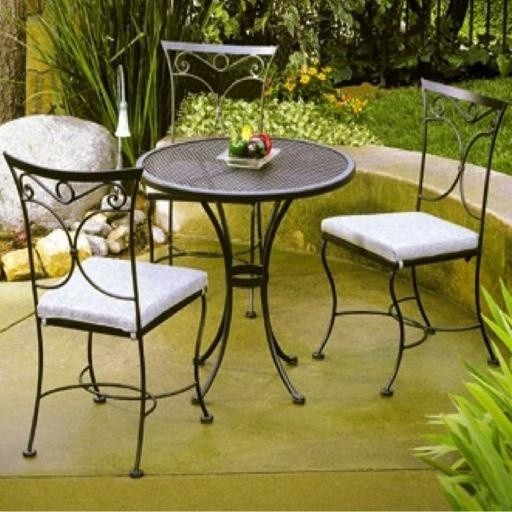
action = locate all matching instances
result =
[104,64,134,209]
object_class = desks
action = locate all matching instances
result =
[135,135,356,406]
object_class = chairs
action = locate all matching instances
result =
[312,78,509,398]
[161,36,280,319]
[2,150,212,478]
